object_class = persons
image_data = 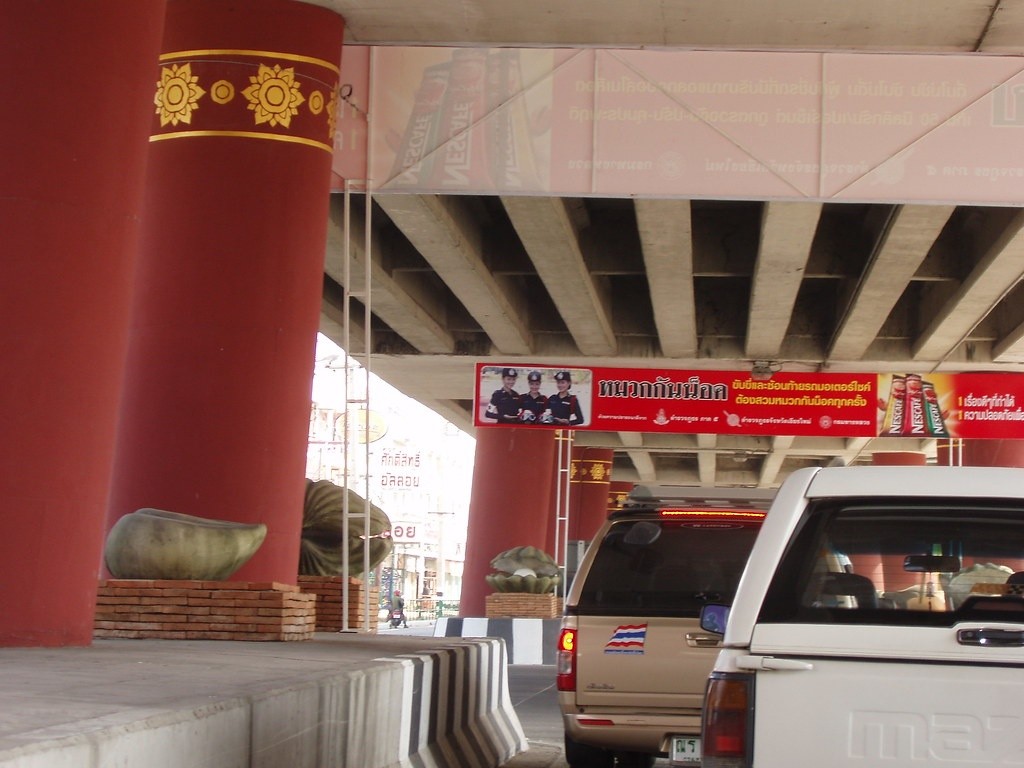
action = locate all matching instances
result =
[484,368,583,425]
[391,590,409,628]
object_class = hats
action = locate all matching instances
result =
[554,371,571,381]
[528,371,541,381]
[502,368,517,377]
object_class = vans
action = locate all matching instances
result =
[699,466,1024,768]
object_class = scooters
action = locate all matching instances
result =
[390,609,401,629]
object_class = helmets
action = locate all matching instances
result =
[393,590,400,596]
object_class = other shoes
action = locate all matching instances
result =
[404,625,408,628]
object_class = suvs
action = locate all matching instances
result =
[555,484,859,768]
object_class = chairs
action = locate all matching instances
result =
[813,572,878,609]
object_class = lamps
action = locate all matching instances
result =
[751,359,781,383]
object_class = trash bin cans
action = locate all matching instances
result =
[419,596,433,612]
